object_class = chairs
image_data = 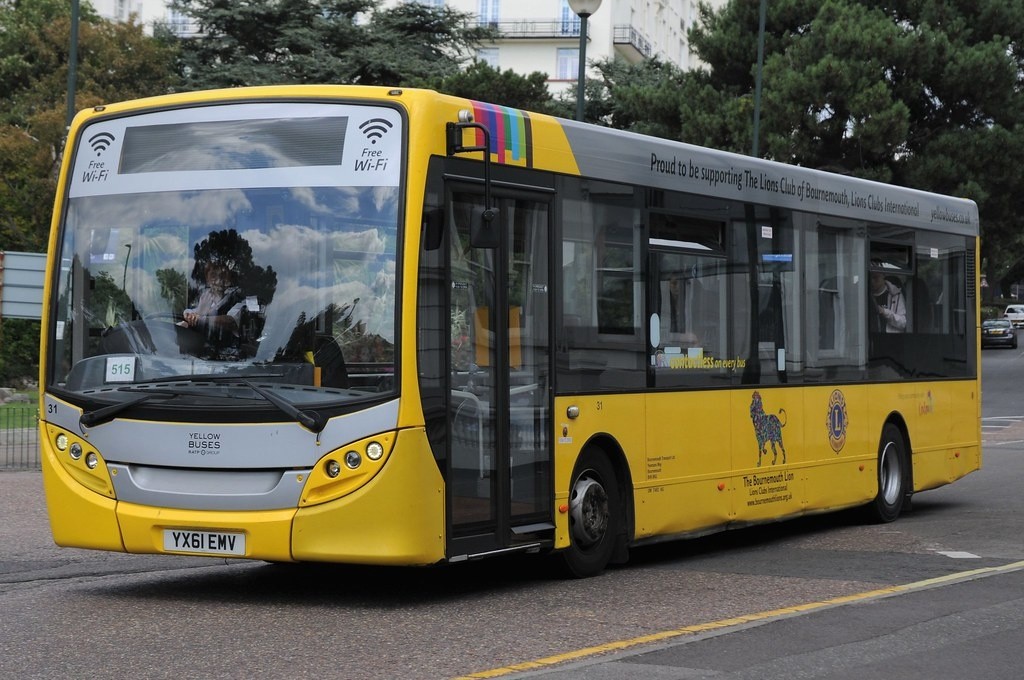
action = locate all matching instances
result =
[902,276,935,335]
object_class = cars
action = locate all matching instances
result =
[982,317,1018,350]
[1004,303,1024,328]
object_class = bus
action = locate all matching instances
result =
[38,84,983,581]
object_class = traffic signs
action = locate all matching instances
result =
[451,281,469,289]
[532,284,548,294]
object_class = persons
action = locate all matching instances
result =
[866,258,907,333]
[175,256,247,345]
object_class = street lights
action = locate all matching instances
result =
[343,298,361,340]
[122,242,133,294]
[564,0,605,122]
